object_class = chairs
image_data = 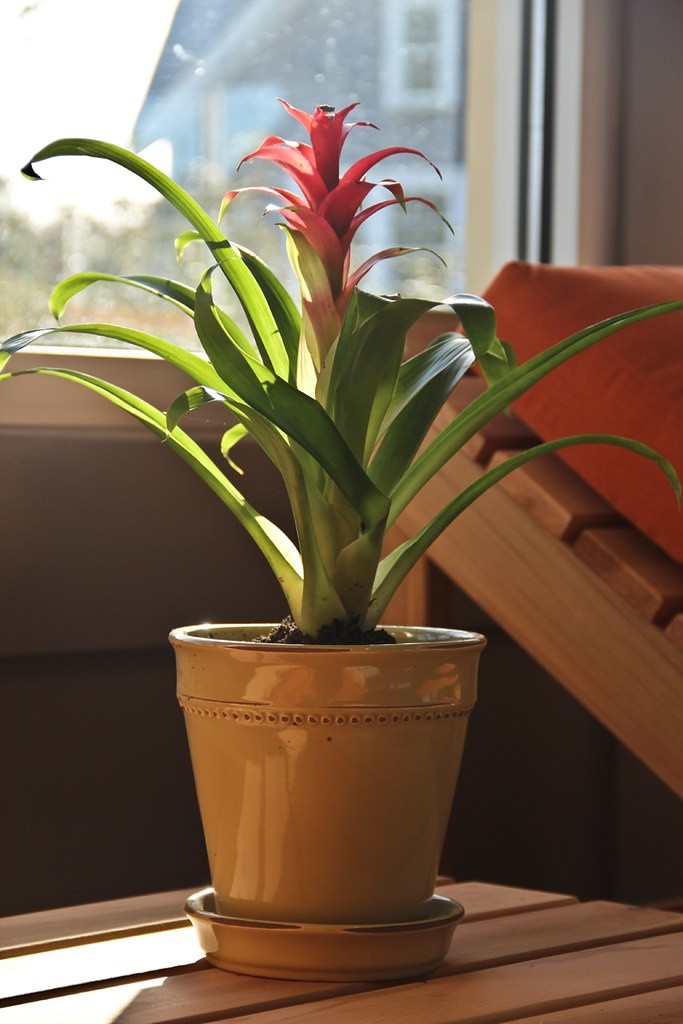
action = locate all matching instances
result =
[379,261,683,795]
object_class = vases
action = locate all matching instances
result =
[164,620,483,982]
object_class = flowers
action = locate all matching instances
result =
[1,100,682,640]
